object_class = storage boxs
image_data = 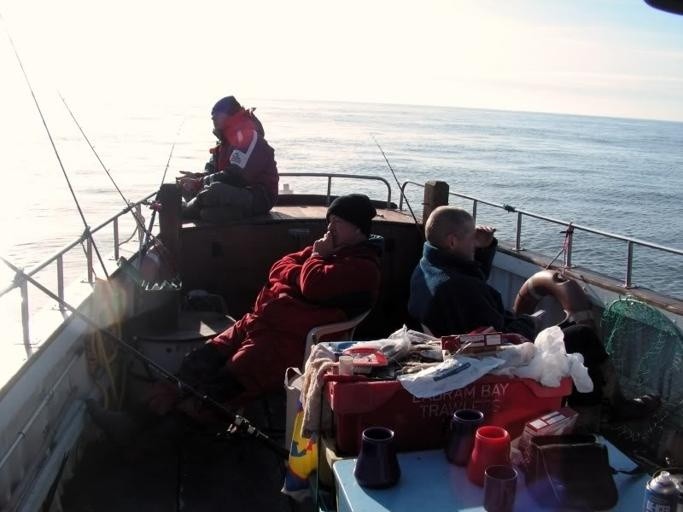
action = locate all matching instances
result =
[324,376,573,457]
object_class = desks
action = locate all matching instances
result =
[279,341,683,512]
[123,310,238,382]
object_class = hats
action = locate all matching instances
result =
[326,194,377,233]
[213,96,241,115]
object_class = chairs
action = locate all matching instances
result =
[420,321,441,338]
[269,307,373,468]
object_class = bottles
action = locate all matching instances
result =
[445,407,486,465]
[467,425,512,487]
[643,471,680,512]
[353,426,402,490]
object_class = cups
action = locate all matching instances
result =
[483,465,519,511]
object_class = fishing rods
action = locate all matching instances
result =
[367,135,418,224]
[7,38,109,278]
[2,256,270,443]
[40,56,158,251]
[138,144,174,271]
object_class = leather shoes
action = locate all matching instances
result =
[85,397,138,448]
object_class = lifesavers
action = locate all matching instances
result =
[513,271,603,336]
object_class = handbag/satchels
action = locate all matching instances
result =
[524,433,618,512]
[280,368,319,503]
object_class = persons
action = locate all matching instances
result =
[176,95,279,223]
[409,205,661,422]
[142,195,381,422]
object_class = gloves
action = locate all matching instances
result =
[175,170,203,193]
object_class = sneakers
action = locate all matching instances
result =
[622,392,661,419]
